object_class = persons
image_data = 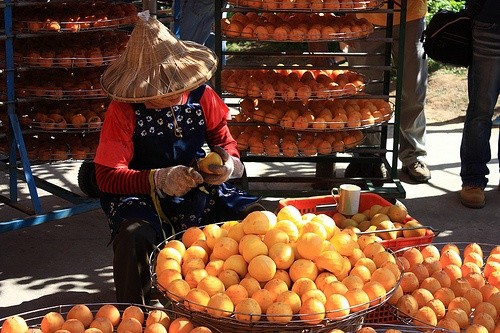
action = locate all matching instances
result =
[172,0,227,90]
[94,10,266,310]
[460,0,500,208]
[348,0,431,183]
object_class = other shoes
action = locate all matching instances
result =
[461,186,486,208]
[402,160,431,180]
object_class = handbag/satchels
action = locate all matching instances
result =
[421,7,473,68]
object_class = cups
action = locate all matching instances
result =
[331,184,361,215]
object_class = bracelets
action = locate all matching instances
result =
[154,168,167,198]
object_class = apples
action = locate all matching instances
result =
[198,152,224,174]
[333,203,427,239]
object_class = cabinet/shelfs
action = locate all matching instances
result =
[0,0,181,233]
[215,0,406,205]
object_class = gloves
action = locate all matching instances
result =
[155,165,203,196]
[204,145,244,184]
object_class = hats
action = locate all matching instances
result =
[101,10,218,102]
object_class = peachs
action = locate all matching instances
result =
[0,2,139,158]
[220,0,392,155]
[385,242,500,333]
[156,205,401,325]
[0,305,213,333]
